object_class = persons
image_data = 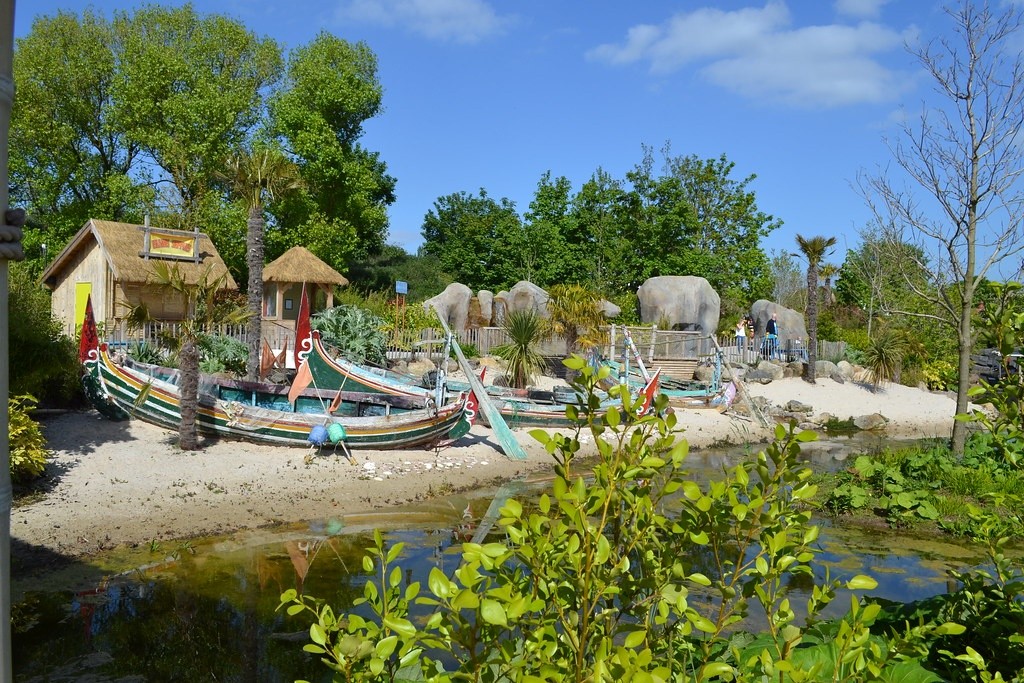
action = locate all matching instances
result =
[766,313,781,361]
[736,319,747,354]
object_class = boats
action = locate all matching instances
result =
[286,278,737,428]
[80,333,488,449]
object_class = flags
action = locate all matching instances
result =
[258,340,276,382]
[288,360,313,404]
[276,338,288,364]
[328,388,342,412]
